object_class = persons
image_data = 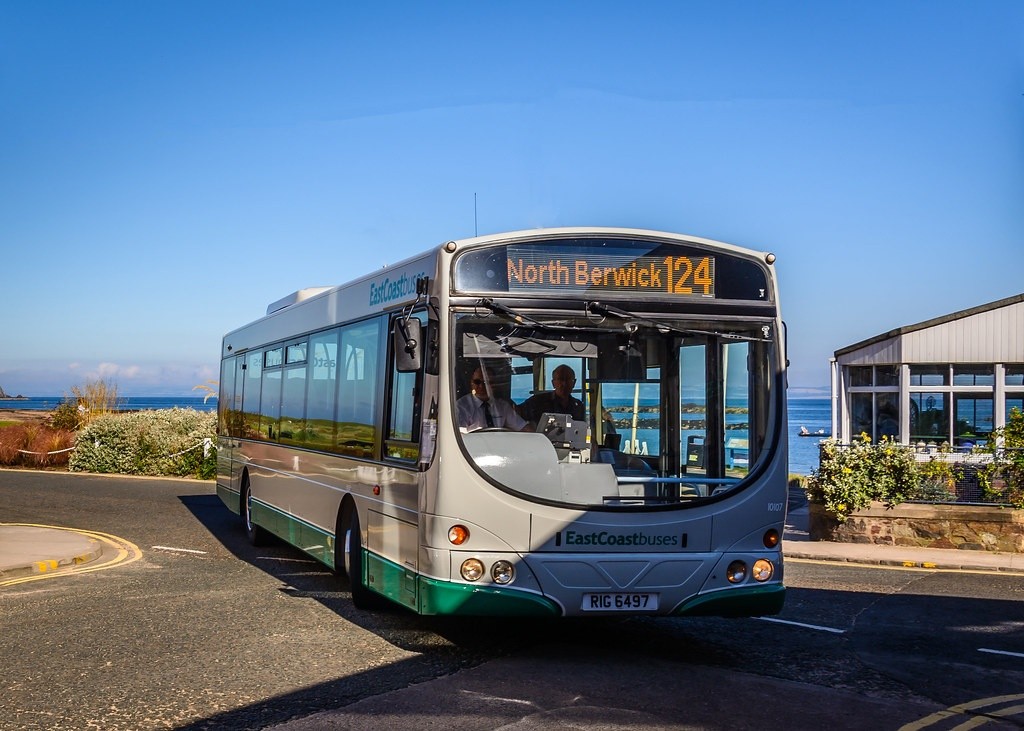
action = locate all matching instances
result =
[801,426,808,434]
[456,367,538,434]
[516,366,587,420]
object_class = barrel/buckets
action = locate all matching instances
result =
[958,433,977,453]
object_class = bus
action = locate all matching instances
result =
[217,227,790,619]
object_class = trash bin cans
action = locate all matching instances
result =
[686,435,707,469]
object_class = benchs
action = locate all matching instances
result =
[243,378,371,424]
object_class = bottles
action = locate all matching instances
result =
[927,440,937,453]
[917,440,926,452]
[942,441,949,452]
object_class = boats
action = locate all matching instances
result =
[798,427,832,436]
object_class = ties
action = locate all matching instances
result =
[482,401,495,428]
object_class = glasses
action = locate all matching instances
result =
[471,378,495,386]
[553,377,575,384]
